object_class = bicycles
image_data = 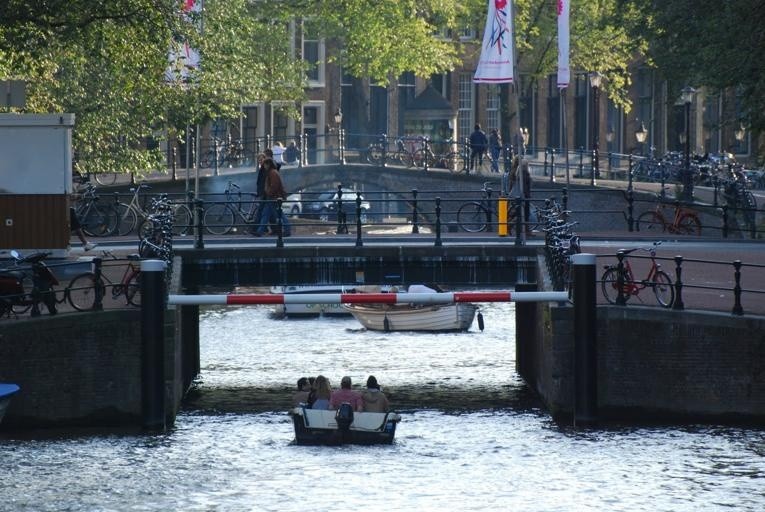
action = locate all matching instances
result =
[200,134,255,171]
[600,240,674,308]
[631,150,765,239]
[366,132,414,169]
[64,157,193,311]
[456,180,540,233]
[538,198,583,289]
[203,181,265,235]
[412,136,466,173]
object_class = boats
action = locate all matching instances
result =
[288,403,403,448]
[269,284,410,317]
[338,282,477,333]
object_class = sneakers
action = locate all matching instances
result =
[250,231,261,236]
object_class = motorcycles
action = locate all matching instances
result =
[0,249,61,319]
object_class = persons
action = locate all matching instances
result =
[508,154,523,235]
[519,128,526,156]
[469,124,489,169]
[69,183,96,252]
[488,128,502,172]
[360,376,392,411]
[240,148,292,238]
[282,142,301,165]
[329,375,362,409]
[306,375,332,409]
[511,159,536,237]
[294,377,311,403]
[271,141,286,166]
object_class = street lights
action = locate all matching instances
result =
[605,123,619,177]
[635,119,649,181]
[333,107,345,165]
[680,85,695,200]
[725,120,746,156]
[587,70,604,180]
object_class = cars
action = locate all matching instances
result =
[277,190,303,219]
[304,187,371,226]
